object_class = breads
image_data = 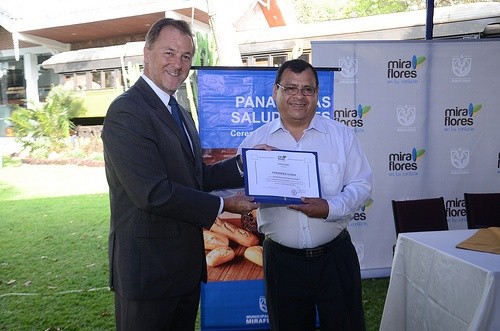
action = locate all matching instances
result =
[203,230,229,249]
[206,246,235,266]
[244,246,263,267]
[241,209,257,234]
[210,217,259,246]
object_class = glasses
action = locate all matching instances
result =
[276,83,318,96]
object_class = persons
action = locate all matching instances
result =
[101,18,280,331]
[236,59,373,331]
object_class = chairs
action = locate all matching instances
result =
[464,193,500,228]
[392,197,449,254]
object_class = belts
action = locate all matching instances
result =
[266,228,348,258]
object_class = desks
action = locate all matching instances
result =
[203,218,264,282]
[380,229,500,331]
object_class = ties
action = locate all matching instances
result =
[168,95,193,154]
